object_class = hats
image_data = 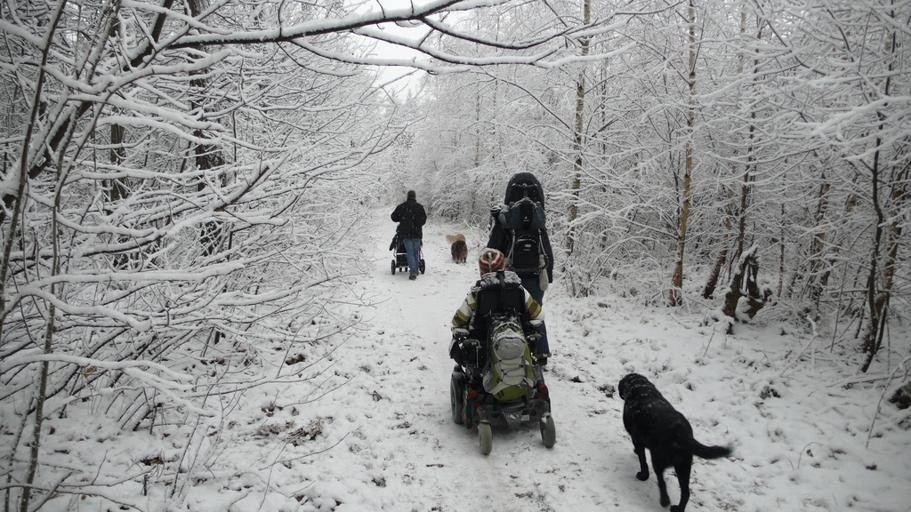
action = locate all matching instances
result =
[479,248,506,275]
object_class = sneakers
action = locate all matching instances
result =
[409,272,419,280]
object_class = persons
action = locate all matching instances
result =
[389,224,409,273]
[486,202,555,368]
[392,189,428,281]
[451,248,543,329]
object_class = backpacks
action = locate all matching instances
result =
[483,310,536,402]
[509,232,540,276]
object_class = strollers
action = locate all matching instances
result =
[391,231,425,274]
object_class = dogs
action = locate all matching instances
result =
[451,240,468,264]
[618,373,732,512]
